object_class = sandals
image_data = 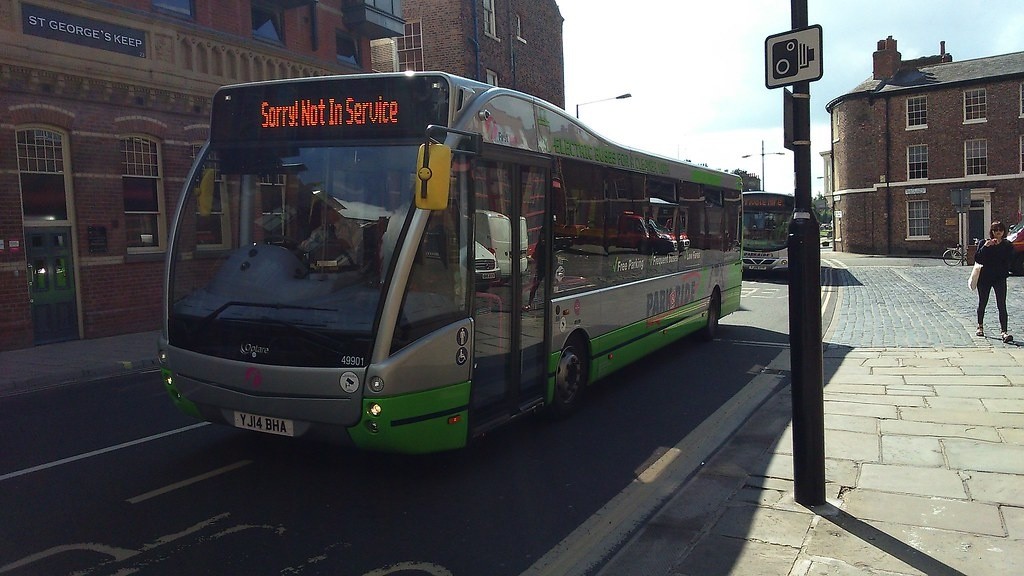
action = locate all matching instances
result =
[1001,332,1013,343]
[976,325,984,336]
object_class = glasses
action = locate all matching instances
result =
[992,228,1004,232]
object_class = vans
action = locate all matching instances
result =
[475,209,530,287]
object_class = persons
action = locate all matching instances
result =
[521,227,546,312]
[296,197,363,266]
[974,221,1014,342]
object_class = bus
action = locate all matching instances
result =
[161,71,744,458]
[22,216,80,343]
[741,192,796,282]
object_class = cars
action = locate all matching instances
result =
[1000,213,1024,275]
[474,239,502,294]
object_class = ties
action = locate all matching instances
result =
[326,225,337,239]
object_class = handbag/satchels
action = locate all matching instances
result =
[967,238,988,291]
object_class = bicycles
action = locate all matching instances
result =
[942,237,980,266]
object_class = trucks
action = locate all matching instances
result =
[553,211,690,256]
